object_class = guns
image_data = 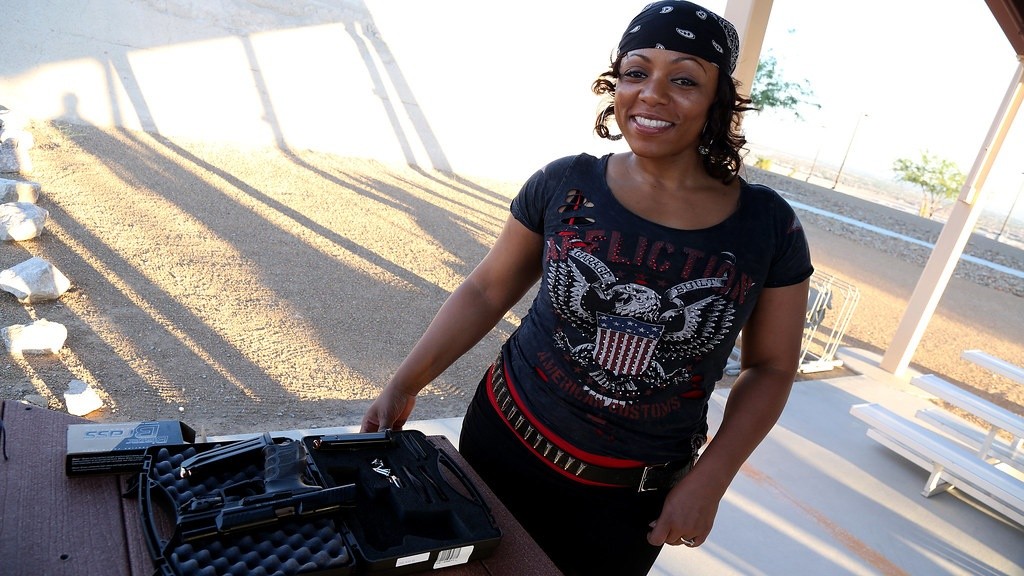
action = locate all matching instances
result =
[174,440,357,540]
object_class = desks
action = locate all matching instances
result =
[1,399,562,576]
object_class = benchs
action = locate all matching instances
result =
[849,348,1024,526]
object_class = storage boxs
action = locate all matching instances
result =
[64,420,196,477]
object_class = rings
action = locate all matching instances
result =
[680,537,694,547]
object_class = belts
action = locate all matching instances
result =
[492,357,698,500]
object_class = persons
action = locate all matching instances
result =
[361,1,814,576]
